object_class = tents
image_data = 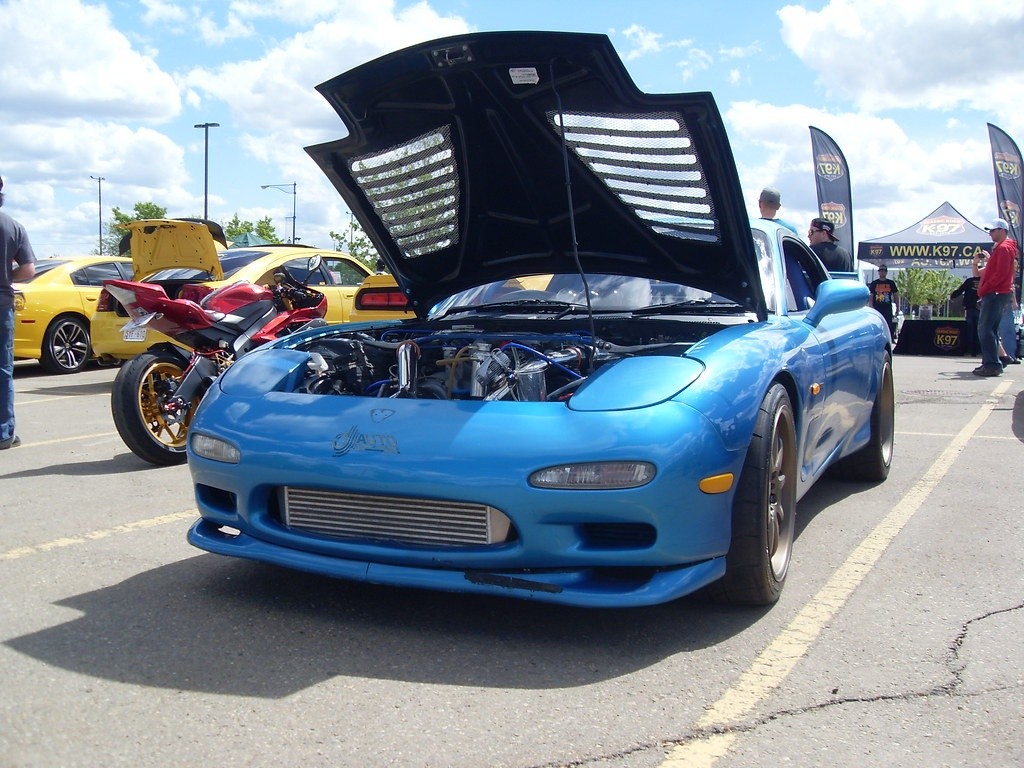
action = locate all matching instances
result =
[856,200,998,285]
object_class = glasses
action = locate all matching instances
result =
[808,229,825,236]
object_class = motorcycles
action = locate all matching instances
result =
[102,254,328,465]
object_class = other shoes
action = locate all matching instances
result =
[973,364,1003,375]
[1013,358,1021,364]
[0,436,21,450]
[999,354,1015,368]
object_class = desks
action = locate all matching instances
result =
[894,320,968,356]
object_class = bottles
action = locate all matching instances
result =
[911,310,915,320]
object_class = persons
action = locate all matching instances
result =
[973,218,1021,377]
[946,267,983,357]
[868,264,900,344]
[758,187,798,237]
[806,215,854,272]
[374,258,389,275]
[0,172,38,450]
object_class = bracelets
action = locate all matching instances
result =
[896,312,898,315]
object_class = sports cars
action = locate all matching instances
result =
[90,218,387,366]
[347,268,555,323]
[9,256,167,373]
[187,32,896,617]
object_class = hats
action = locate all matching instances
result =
[984,218,1009,231]
[878,264,887,272]
[812,218,839,241]
[760,187,780,204]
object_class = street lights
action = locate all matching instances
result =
[260,184,297,246]
[194,122,221,221]
[346,213,355,246]
[92,175,106,256]
